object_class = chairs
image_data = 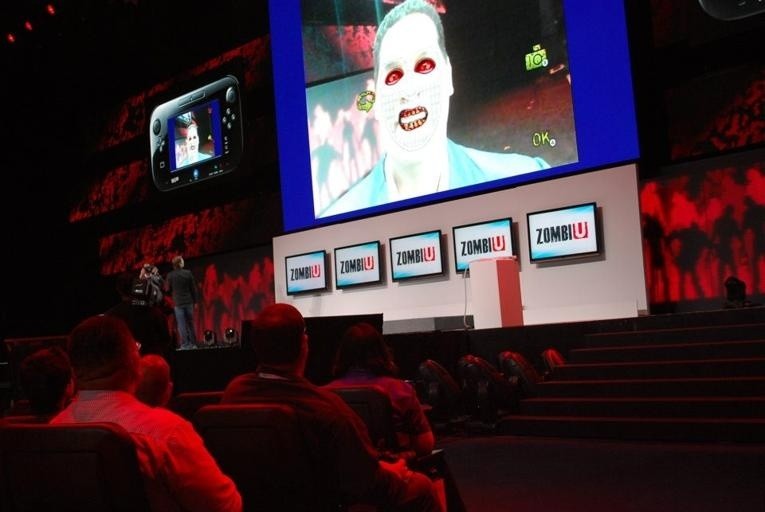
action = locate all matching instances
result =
[0,377,444,512]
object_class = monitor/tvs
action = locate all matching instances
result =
[451,217,515,273]
[284,249,328,296]
[526,202,601,264]
[388,229,445,281]
[334,240,383,290]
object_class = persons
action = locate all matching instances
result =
[642,195,765,302]
[2,313,241,512]
[317,0,551,219]
[165,256,199,349]
[223,303,448,512]
[178,124,213,167]
